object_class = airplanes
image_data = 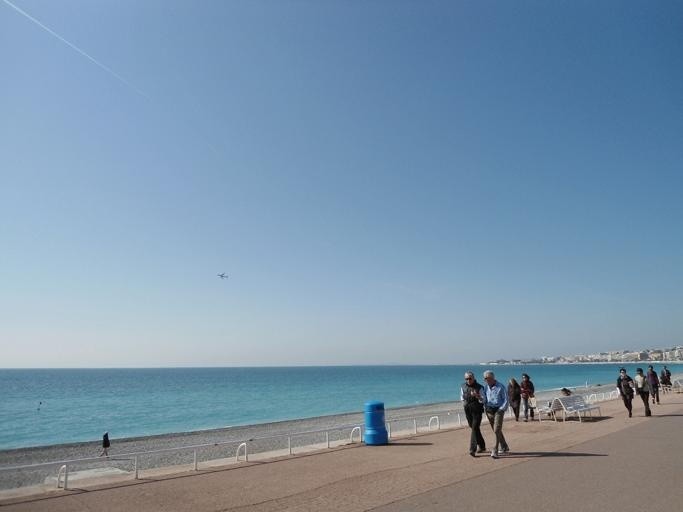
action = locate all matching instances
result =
[217,273,228,279]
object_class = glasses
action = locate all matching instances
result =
[465,377,472,381]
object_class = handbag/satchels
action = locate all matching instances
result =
[527,396,537,409]
[636,387,644,395]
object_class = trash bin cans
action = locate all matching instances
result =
[363,400,388,446]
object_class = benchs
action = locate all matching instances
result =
[658,378,682,394]
[536,397,561,421]
[555,396,603,424]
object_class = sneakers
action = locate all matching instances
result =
[469,450,475,457]
[490,447,499,459]
[498,445,510,454]
[523,418,528,422]
[477,447,486,453]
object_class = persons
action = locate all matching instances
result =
[616,367,634,418]
[519,372,535,422]
[480,369,509,458]
[98,430,111,459]
[507,377,521,421]
[645,364,659,405]
[547,386,572,417]
[459,371,485,458]
[633,367,651,417]
[661,365,671,394]
